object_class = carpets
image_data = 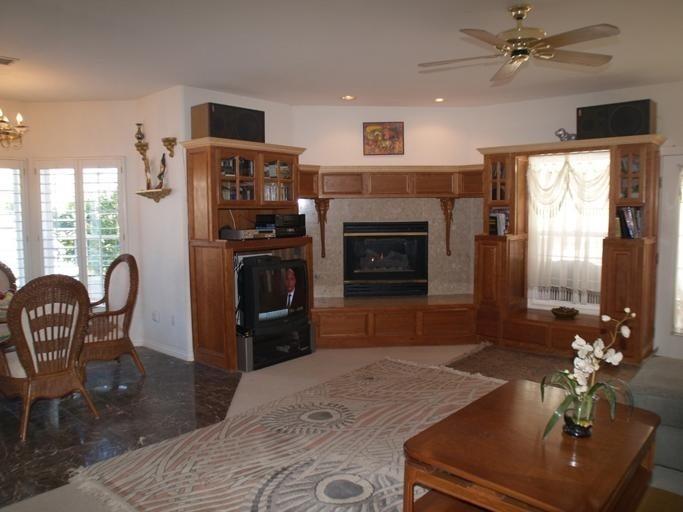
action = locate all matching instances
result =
[66,357,508,512]
[443,343,639,397]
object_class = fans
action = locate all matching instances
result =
[417,5,620,81]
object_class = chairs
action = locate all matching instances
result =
[76,254,146,384]
[0,275,101,440]
[0,261,17,349]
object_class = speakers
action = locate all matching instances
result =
[576,99,657,140]
[190,102,265,143]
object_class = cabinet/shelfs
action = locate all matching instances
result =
[472,143,661,361]
[183,138,313,372]
[299,164,481,198]
[313,296,479,344]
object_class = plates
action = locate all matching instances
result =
[551,308,578,318]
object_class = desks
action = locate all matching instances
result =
[402,380,661,511]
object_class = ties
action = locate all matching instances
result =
[287,294,292,307]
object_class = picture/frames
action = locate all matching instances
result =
[363,122,405,156]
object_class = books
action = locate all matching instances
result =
[221,158,253,176]
[614,205,640,240]
[264,182,292,201]
[222,181,253,199]
[489,205,510,237]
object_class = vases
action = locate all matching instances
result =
[134,122,145,144]
[567,395,596,437]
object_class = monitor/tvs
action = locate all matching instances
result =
[238,258,308,330]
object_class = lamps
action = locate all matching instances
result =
[0,109,30,149]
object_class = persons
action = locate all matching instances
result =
[282,267,302,313]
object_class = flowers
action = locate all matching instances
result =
[539,305,637,441]
